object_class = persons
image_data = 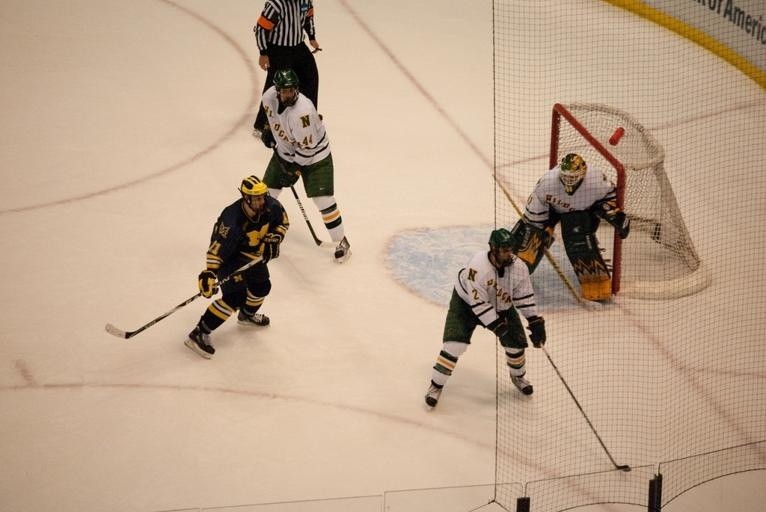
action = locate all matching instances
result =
[184,175,289,360]
[425,229,546,412]
[511,154,631,303]
[252,0,322,138]
[254,69,352,264]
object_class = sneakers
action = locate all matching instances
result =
[334,236,350,263]
[188,321,215,354]
[510,373,533,395]
[253,129,264,138]
[238,310,269,326]
[426,383,443,407]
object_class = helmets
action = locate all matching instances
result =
[240,175,268,196]
[559,154,587,196]
[488,228,518,267]
[273,69,298,107]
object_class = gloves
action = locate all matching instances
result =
[261,130,277,148]
[198,268,218,299]
[614,211,630,239]
[260,231,283,265]
[492,318,528,348]
[279,161,302,187]
[527,317,546,348]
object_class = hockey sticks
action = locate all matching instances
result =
[106,256,267,340]
[538,340,630,470]
[271,142,345,248]
[491,174,602,311]
